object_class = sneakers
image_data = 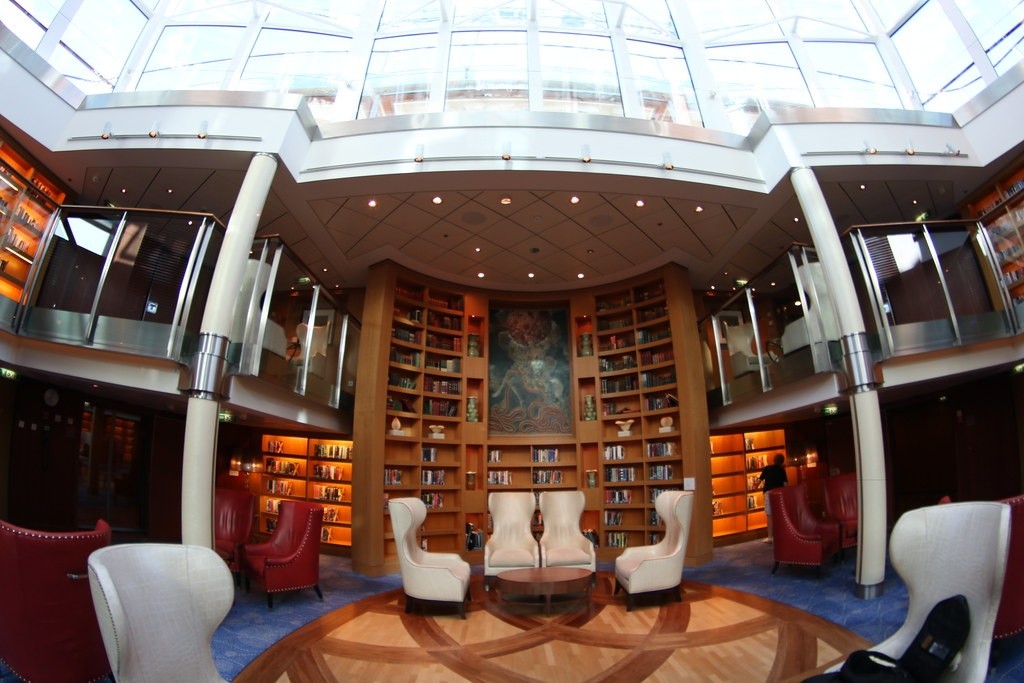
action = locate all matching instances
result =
[763,538,772,543]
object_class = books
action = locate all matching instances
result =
[977,178,1024,288]
[265,278,768,550]
[0,164,52,272]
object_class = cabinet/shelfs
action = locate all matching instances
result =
[350,257,713,574]
[257,428,354,554]
[966,152,1024,309]
[0,125,72,309]
[707,423,790,548]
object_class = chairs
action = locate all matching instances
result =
[87,542,234,683]
[387,497,472,620]
[237,501,324,608]
[214,486,259,582]
[768,483,840,580]
[823,500,1012,683]
[484,493,540,592]
[614,490,694,612]
[779,260,840,358]
[286,322,332,378]
[536,489,597,590]
[0,517,113,683]
[989,494,1024,675]
[722,320,778,378]
[820,472,859,561]
[230,259,287,358]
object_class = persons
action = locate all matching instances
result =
[753,454,788,544]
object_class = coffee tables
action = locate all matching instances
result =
[497,566,592,617]
